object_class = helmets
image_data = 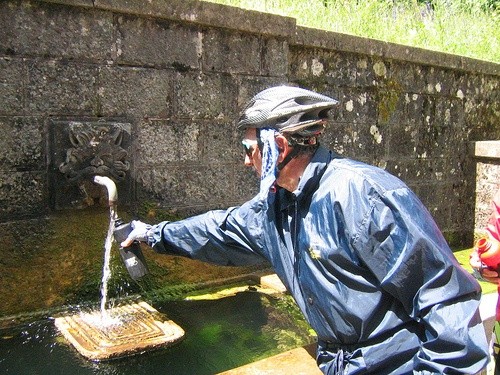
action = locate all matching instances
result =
[238,84,341,149]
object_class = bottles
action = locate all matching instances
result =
[476,238,500,278]
[113,218,148,279]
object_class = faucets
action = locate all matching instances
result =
[94,176,118,205]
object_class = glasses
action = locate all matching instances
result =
[240,138,261,158]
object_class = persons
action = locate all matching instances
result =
[469,192,500,375]
[120,86,491,375]
